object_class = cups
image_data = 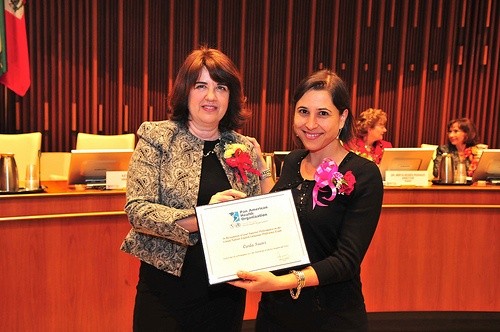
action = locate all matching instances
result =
[455,164,466,183]
[25,163,39,191]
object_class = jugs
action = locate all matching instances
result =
[0,153,20,191]
[264,155,274,181]
[440,150,454,183]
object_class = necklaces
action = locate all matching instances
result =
[203,131,222,157]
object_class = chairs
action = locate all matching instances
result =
[0,132,42,189]
[76,132,135,151]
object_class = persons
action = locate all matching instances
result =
[121,48,264,332]
[431,119,487,177]
[226,68,384,331]
[344,107,393,167]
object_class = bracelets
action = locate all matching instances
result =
[258,168,272,181]
[297,271,305,288]
[288,269,302,299]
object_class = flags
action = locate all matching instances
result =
[0,0,31,97]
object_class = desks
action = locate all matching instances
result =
[0,181,500,332]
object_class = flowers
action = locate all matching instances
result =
[223,143,251,160]
[333,170,357,195]
